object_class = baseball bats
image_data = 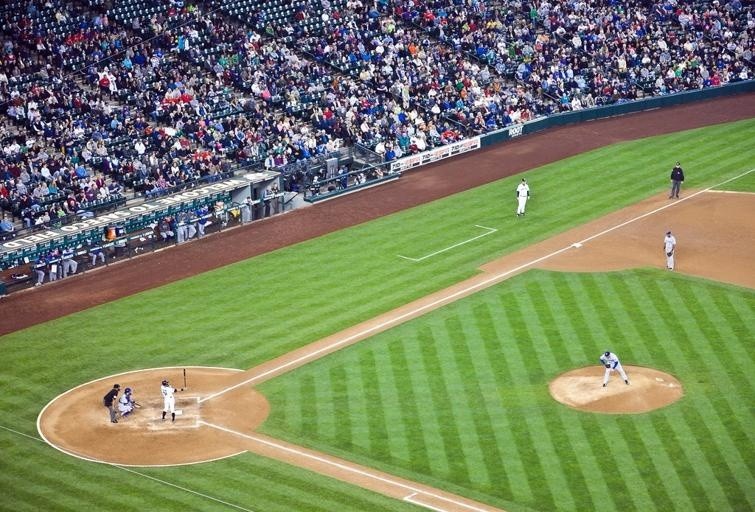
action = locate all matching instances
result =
[184,369,186,388]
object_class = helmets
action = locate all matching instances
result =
[125,388,131,392]
[162,380,168,385]
[113,384,120,388]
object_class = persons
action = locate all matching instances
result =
[118,387,135,418]
[161,380,188,423]
[664,231,677,270]
[516,178,532,217]
[600,351,630,388]
[104,383,121,423]
[669,162,685,199]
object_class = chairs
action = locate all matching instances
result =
[0,0,755,238]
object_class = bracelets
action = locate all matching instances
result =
[180,388,184,391]
[672,249,673,251]
[664,247,665,249]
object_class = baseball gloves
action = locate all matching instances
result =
[133,401,141,408]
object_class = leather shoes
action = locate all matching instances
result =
[113,420,118,423]
